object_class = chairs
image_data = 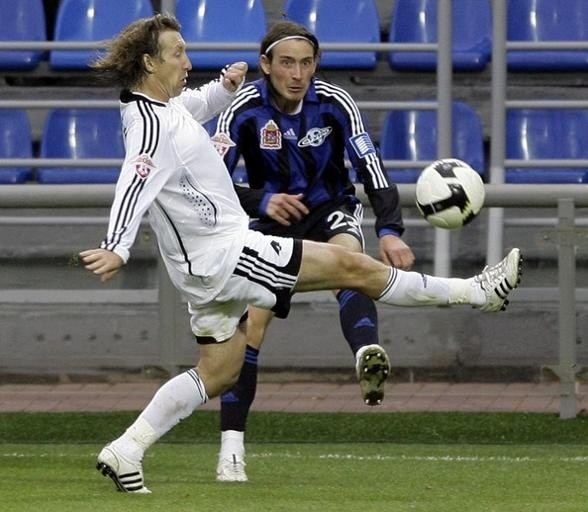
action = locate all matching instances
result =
[0,0,588,184]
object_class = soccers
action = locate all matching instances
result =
[416,159,485,229]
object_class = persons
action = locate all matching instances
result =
[77,12,526,494]
[211,19,416,483]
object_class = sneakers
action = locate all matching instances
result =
[354,342,391,407]
[216,446,248,483]
[97,441,151,495]
[475,247,526,313]
